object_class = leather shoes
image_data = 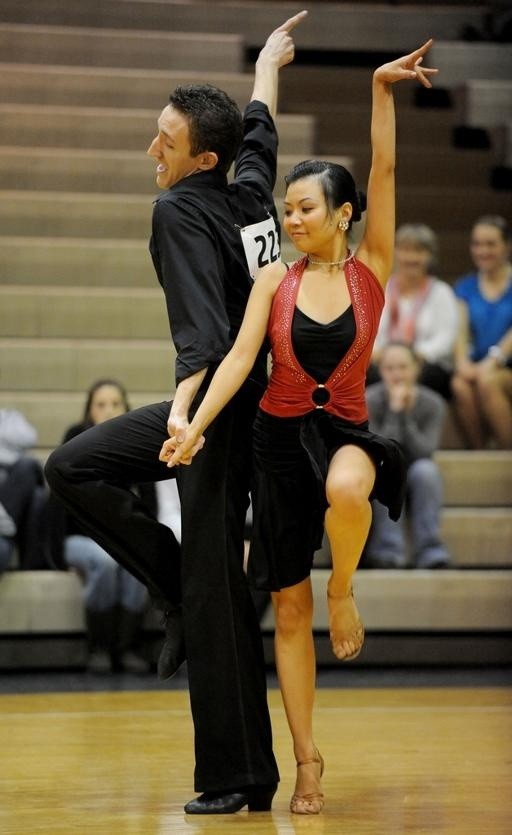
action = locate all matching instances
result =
[156,607,187,681]
[184,783,277,813]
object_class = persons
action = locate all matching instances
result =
[364,221,461,449]
[44,7,310,816]
[47,378,156,678]
[157,37,438,818]
[452,213,512,450]
[363,339,450,569]
[0,406,38,575]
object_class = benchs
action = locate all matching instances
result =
[0,0,512,671]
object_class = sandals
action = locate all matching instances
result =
[327,580,365,662]
[290,749,324,813]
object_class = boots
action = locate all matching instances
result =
[85,605,152,674]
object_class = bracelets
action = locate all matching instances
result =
[486,344,508,368]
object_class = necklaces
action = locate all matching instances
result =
[307,250,354,266]
